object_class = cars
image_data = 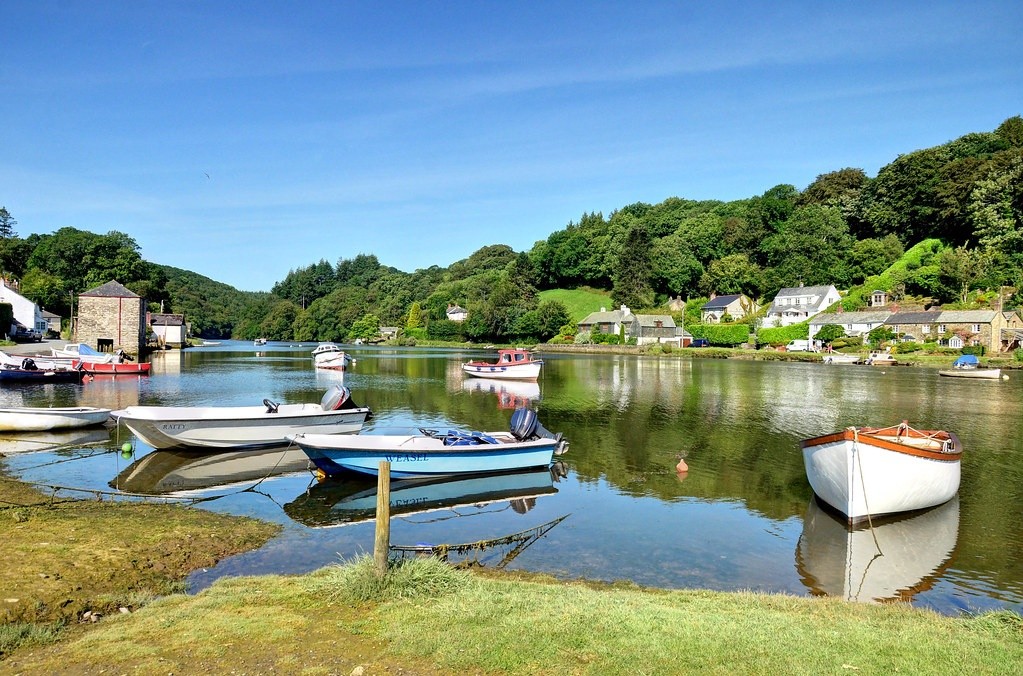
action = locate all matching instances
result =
[13,328,42,343]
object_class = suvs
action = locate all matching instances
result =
[687,339,710,347]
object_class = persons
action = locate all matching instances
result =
[828,343,832,354]
[869,351,877,358]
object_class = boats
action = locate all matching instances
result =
[282,469,559,530]
[311,344,351,370]
[939,364,1001,379]
[51,344,151,375]
[795,492,964,602]
[0,406,113,432]
[822,354,860,364]
[464,375,540,408]
[799,419,963,526]
[286,405,568,480]
[0,427,111,453]
[254,338,266,347]
[110,384,374,449]
[462,347,544,380]
[952,354,979,369]
[0,347,86,383]
[108,447,320,501]
[865,350,897,366]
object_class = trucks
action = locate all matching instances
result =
[786,340,821,352]
